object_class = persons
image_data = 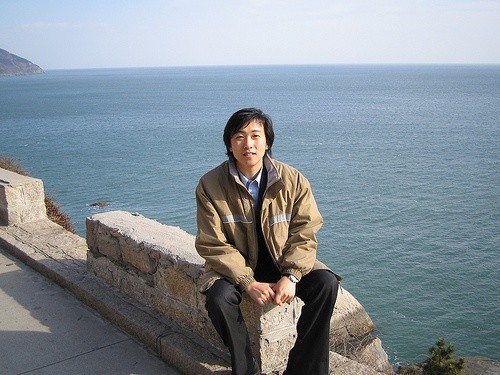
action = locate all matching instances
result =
[195,108,344,375]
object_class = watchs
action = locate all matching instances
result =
[286,274,299,283]
